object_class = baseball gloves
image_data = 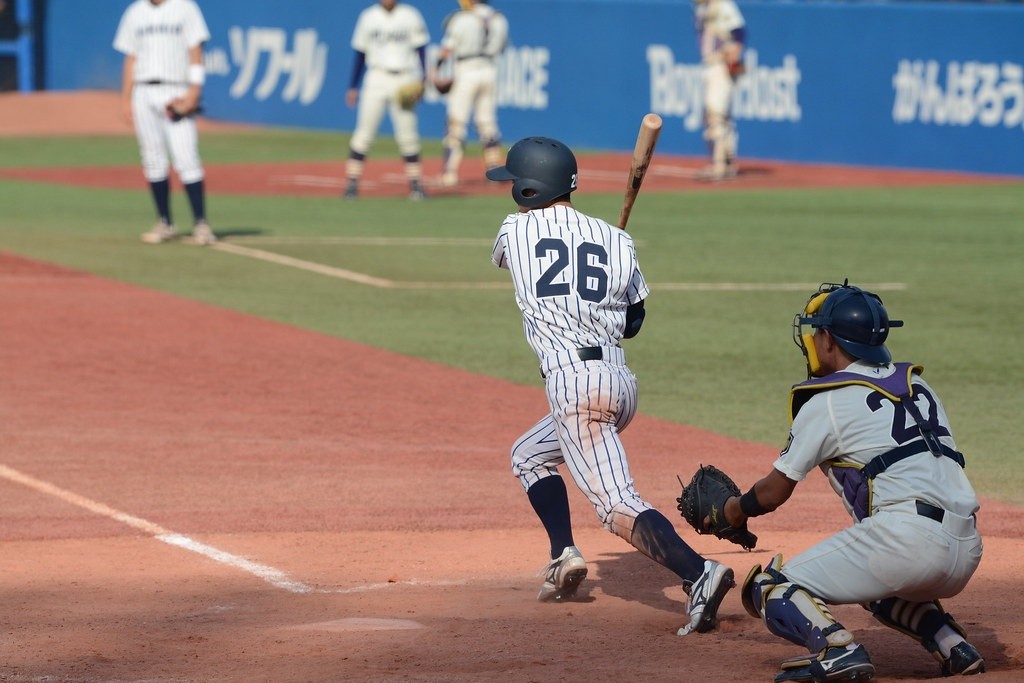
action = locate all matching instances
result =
[674,462,759,554]
[398,80,425,109]
[725,53,747,82]
[436,78,452,94]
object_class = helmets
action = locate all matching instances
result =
[484,138,578,210]
[818,288,891,367]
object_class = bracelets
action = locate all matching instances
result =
[188,64,206,86]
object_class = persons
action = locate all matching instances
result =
[427,0,508,188]
[486,136,735,636]
[344,0,430,199]
[693,0,746,179]
[675,278,984,683]
[114,0,214,245]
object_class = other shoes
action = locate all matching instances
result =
[344,183,359,198]
[410,184,426,199]
[693,161,739,182]
[439,170,458,188]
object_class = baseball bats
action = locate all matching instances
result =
[618,112,663,231]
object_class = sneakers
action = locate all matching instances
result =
[774,643,875,683]
[141,224,177,245]
[938,642,986,677]
[191,220,216,245]
[535,546,588,603]
[676,559,737,636]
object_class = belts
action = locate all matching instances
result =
[145,79,160,85]
[456,54,495,62]
[540,347,605,378]
[916,501,976,527]
